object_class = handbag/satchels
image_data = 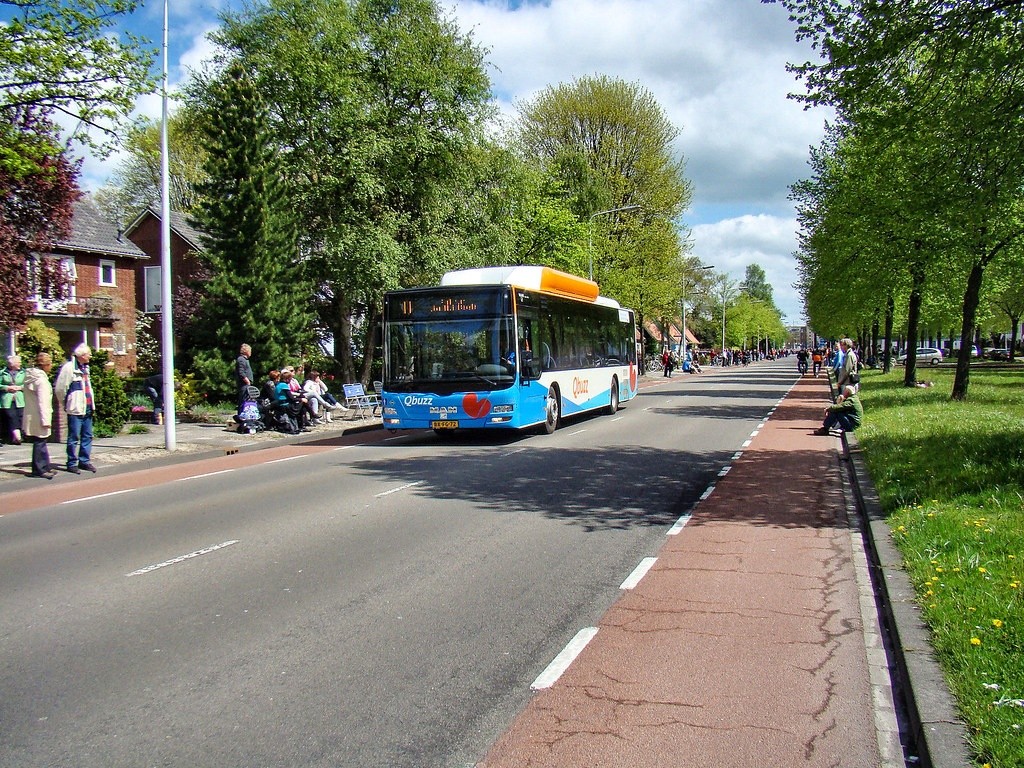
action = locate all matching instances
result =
[850,374,859,383]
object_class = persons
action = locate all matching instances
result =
[812,345,823,378]
[236,343,254,415]
[260,366,349,426]
[0,355,25,444]
[796,345,809,373]
[21,353,57,479]
[824,338,903,429]
[662,350,680,378]
[813,385,863,434]
[682,345,798,374]
[55,343,97,474]
[638,354,642,375]
[487,334,516,375]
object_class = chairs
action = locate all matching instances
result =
[373,381,383,413]
[542,342,603,369]
[343,383,383,422]
[247,386,334,427]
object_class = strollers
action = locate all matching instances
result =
[232,385,266,434]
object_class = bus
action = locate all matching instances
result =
[373,265,639,434]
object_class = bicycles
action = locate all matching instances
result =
[798,360,806,377]
[645,355,662,373]
[814,361,821,379]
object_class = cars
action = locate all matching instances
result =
[972,345,1008,360]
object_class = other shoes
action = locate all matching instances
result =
[50,468,58,474]
[42,472,53,478]
[300,426,311,432]
[12,439,22,445]
[305,421,314,426]
[78,460,97,472]
[67,466,81,474]
[283,423,292,432]
[313,414,322,418]
[814,427,829,435]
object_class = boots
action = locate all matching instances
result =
[313,411,322,424]
[326,411,333,423]
[325,401,336,411]
[334,401,349,412]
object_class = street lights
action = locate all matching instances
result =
[682,264,715,361]
[722,286,749,351]
[589,204,642,281]
[743,300,789,356]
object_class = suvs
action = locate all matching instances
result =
[896,348,942,365]
